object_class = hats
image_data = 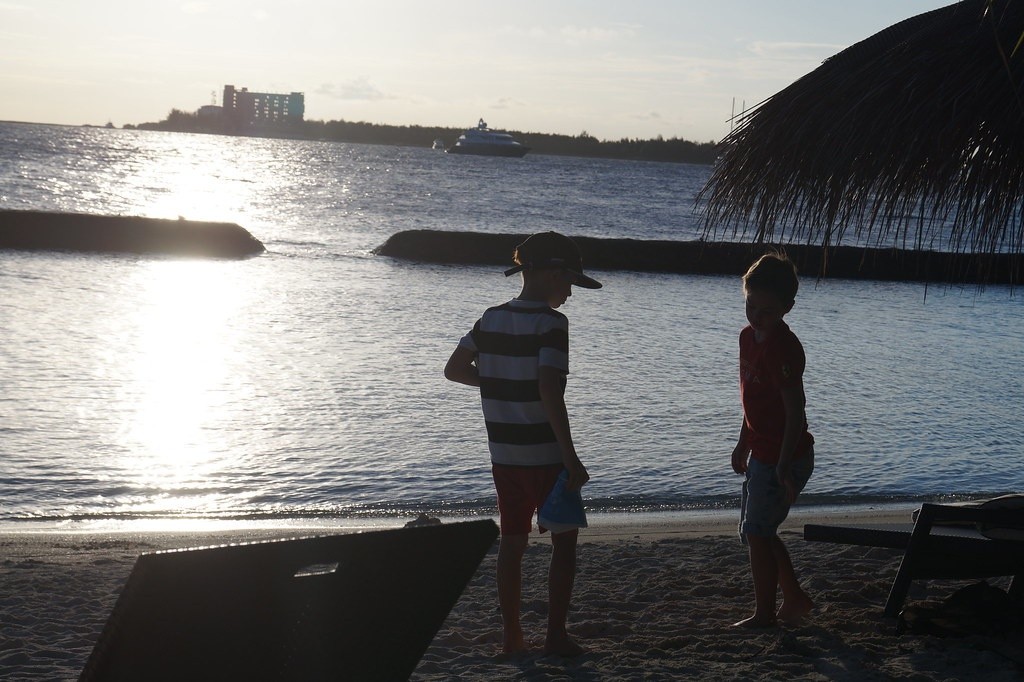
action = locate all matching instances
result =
[505,254,602,289]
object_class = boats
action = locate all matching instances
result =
[446,117,533,158]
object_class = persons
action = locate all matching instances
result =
[722,254,816,628]
[443,230,600,656]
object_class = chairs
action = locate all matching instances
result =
[802,494,1024,621]
[81,520,498,682]
